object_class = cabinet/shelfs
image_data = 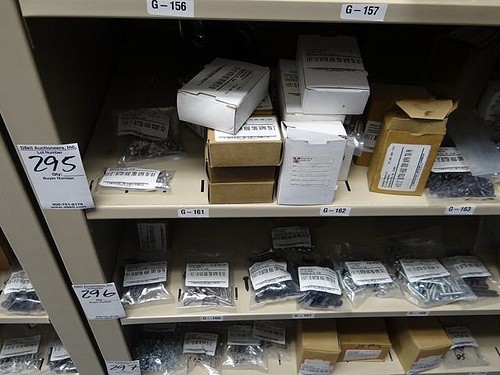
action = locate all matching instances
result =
[0,0,500,375]
